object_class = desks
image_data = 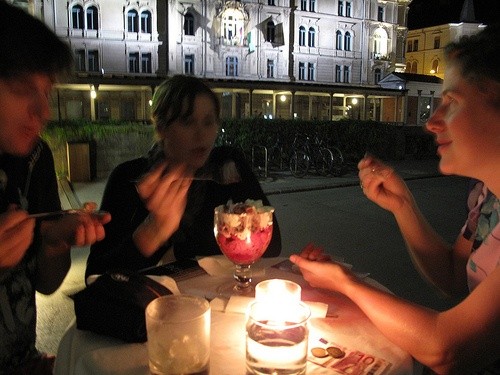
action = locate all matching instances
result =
[52,256,423,375]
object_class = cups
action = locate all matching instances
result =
[255,279,302,300]
[145,294,212,375]
[246,300,312,375]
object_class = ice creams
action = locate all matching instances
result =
[213,198,272,265]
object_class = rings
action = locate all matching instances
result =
[360,182,367,190]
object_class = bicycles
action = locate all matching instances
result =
[220,128,345,179]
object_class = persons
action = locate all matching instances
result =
[0,0,112,375]
[290,25,500,375]
[85,74,282,287]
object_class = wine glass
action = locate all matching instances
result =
[214,203,274,298]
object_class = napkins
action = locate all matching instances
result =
[198,257,265,277]
[209,295,329,319]
